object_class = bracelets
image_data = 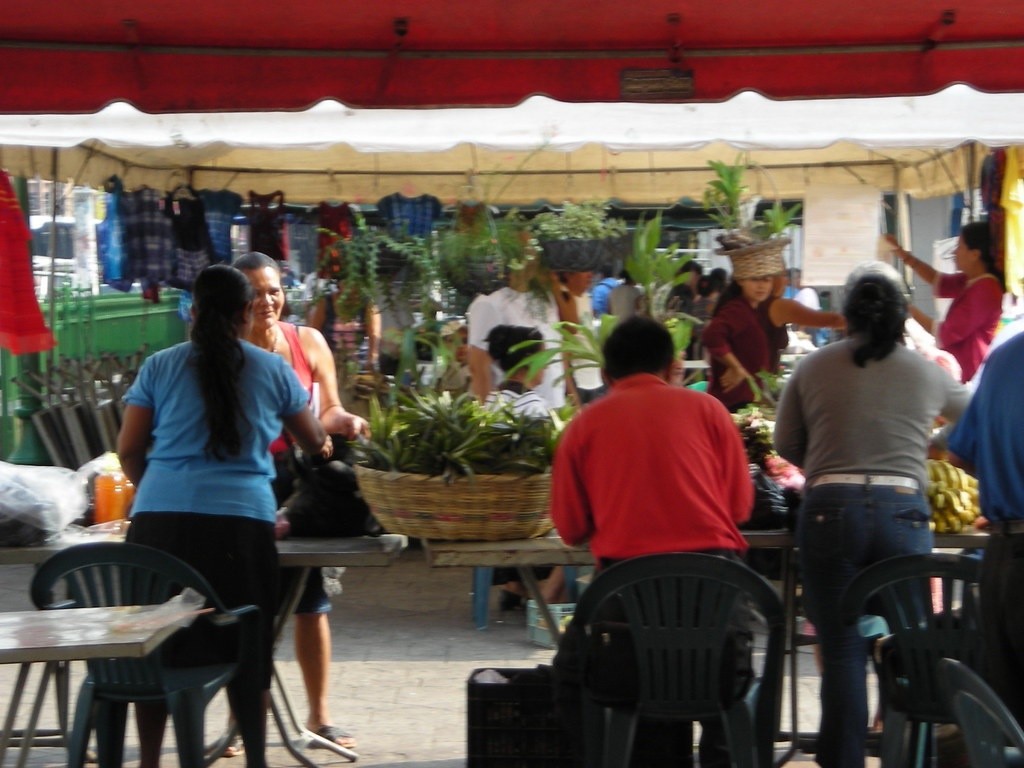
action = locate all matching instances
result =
[891,246,919,267]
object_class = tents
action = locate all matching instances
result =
[0,85,1024,397]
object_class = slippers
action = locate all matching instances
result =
[309,725,358,749]
[222,737,245,755]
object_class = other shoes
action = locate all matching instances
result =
[500,590,528,609]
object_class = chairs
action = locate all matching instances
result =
[29,542,241,768]
[537,552,1024,768]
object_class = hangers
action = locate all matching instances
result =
[304,178,359,212]
[171,169,196,197]
[441,185,501,215]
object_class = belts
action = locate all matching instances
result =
[811,470,921,490]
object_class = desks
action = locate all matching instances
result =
[419,519,991,750]
[0,534,407,768]
[12,341,151,471]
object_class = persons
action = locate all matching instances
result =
[536,248,728,402]
[778,261,973,768]
[759,272,846,373]
[483,325,581,612]
[549,318,755,767]
[118,265,327,767]
[784,267,829,347]
[948,332,1024,733]
[886,222,1003,385]
[701,239,770,412]
[468,231,580,422]
[215,252,373,755]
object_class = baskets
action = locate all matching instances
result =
[353,458,555,539]
[524,598,578,648]
[714,235,790,278]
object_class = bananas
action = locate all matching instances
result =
[925,457,986,534]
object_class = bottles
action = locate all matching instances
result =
[92,458,136,527]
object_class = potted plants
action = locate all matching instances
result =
[315,159,803,316]
